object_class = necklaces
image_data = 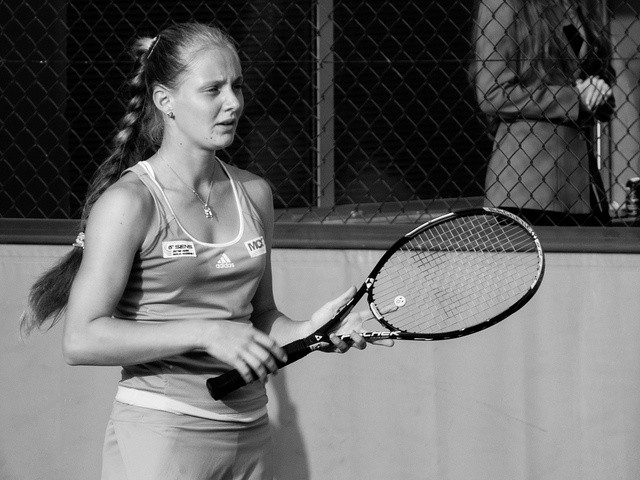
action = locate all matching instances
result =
[157,147,217,218]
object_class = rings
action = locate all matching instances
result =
[601,91,606,96]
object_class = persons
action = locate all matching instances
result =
[475,0,617,226]
[19,22,397,480]
